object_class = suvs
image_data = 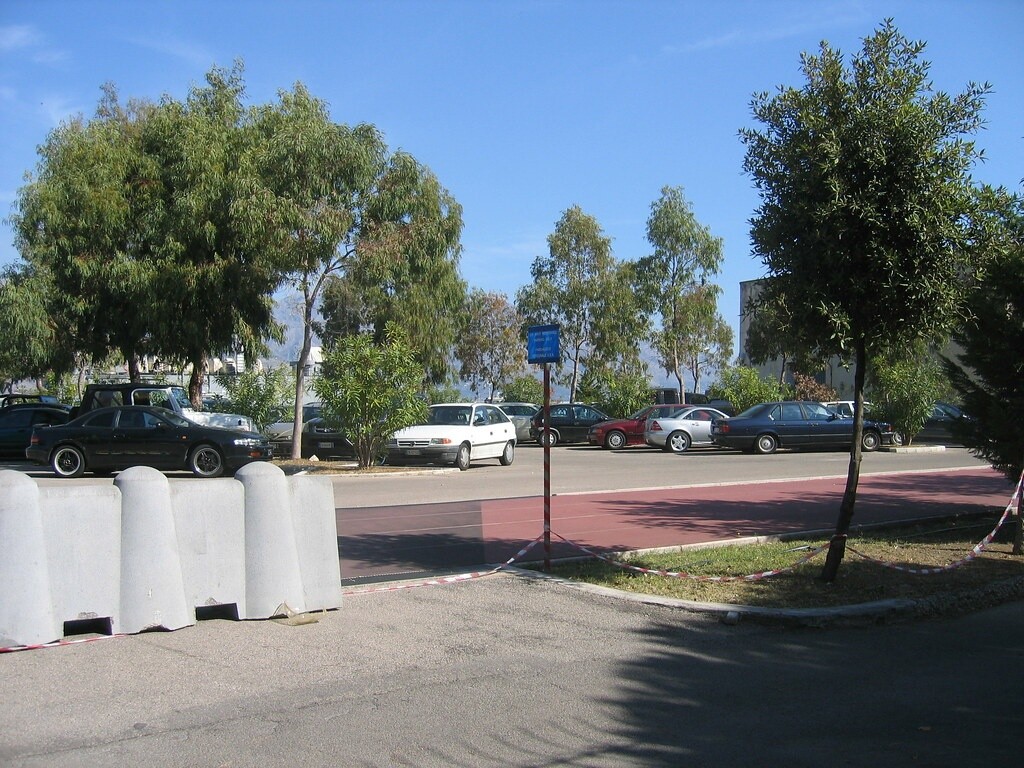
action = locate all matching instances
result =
[69,384,261,446]
[302,394,429,466]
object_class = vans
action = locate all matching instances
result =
[816,401,913,447]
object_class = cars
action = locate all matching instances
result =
[25,405,273,478]
[0,402,72,460]
[643,407,730,452]
[528,404,617,446]
[914,400,980,447]
[264,401,338,454]
[587,404,713,450]
[384,402,519,471]
[707,399,895,454]
[493,402,541,442]
